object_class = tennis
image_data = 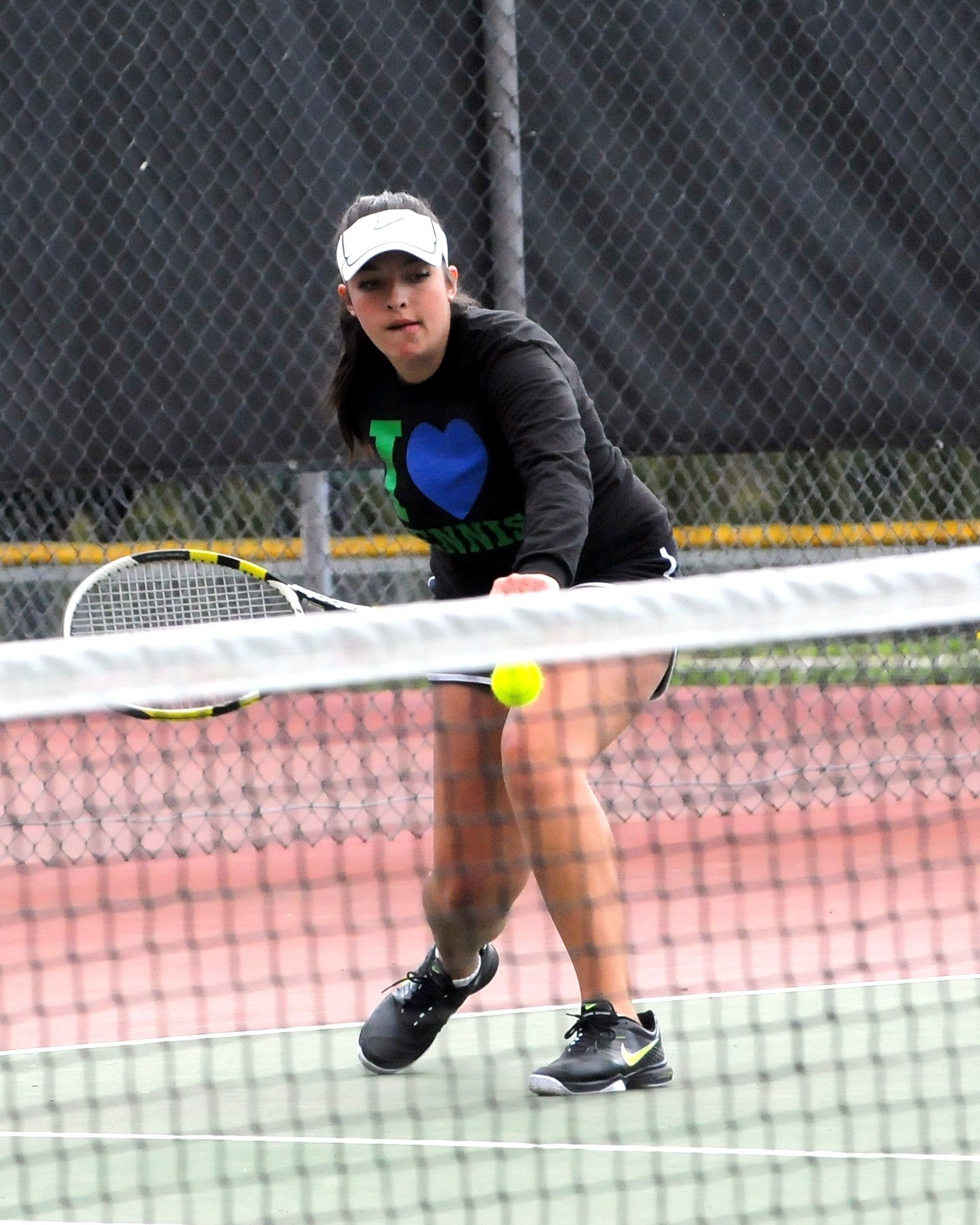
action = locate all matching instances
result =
[490,661,544,706]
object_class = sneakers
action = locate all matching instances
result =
[527,1000,673,1097]
[355,940,500,1075]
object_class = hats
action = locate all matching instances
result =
[335,208,448,285]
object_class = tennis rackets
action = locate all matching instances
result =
[63,550,491,721]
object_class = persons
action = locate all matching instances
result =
[333,192,681,1096]
